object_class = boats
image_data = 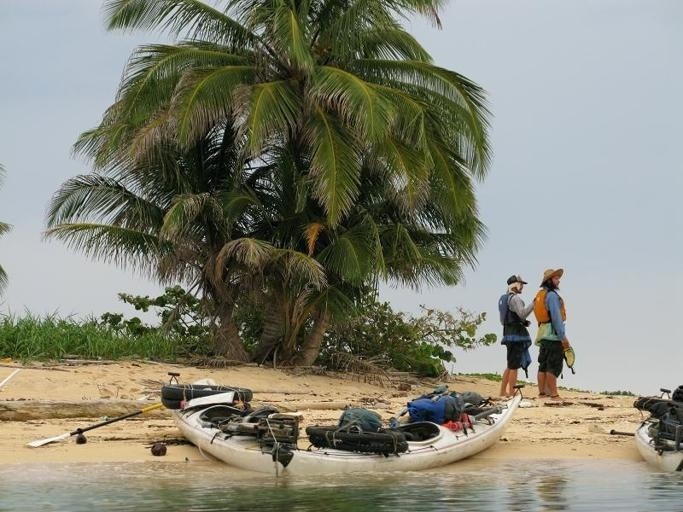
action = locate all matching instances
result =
[175,380,522,475]
[635,390,683,470]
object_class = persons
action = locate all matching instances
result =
[496,275,536,397]
[534,267,575,404]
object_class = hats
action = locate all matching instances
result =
[507,274,528,286]
[539,268,564,287]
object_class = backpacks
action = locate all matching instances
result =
[407,392,483,423]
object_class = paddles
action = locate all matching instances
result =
[587,423,633,435]
[27,402,163,448]
